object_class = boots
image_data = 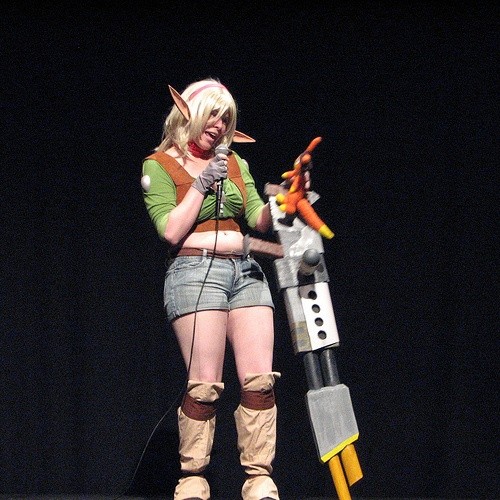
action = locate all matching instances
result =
[234,371,282,500]
[172,380,225,500]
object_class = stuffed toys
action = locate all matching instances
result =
[275,136,335,240]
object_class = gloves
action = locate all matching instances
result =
[192,154,227,194]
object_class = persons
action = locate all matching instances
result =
[142,76,309,500]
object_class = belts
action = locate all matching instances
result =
[171,248,245,259]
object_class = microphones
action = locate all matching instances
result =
[214,144,228,216]
